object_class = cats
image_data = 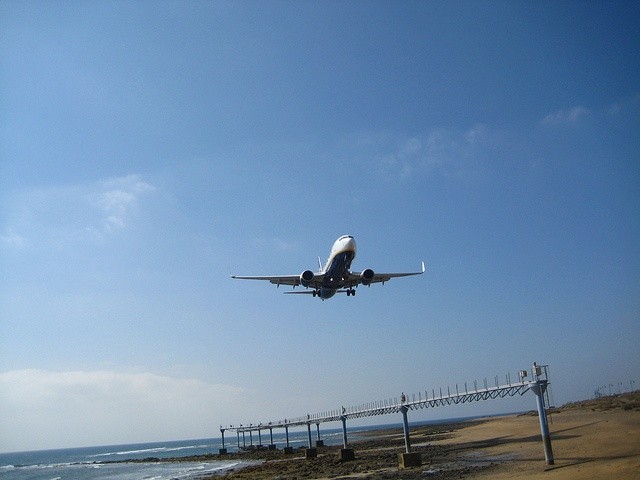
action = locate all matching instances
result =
[228,234,426,300]
